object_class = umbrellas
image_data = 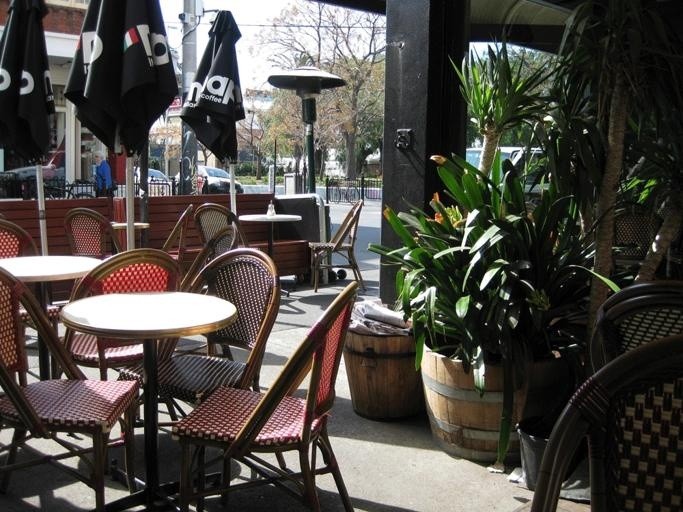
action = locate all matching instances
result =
[63,0,180,252]
[0,0,56,257]
[180,10,246,232]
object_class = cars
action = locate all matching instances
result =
[174,165,245,196]
[133,165,171,184]
[1,150,65,186]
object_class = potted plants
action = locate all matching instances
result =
[368,143,620,466]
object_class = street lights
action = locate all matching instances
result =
[264,65,346,194]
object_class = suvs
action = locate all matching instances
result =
[466,147,553,196]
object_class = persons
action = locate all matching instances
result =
[92,150,113,198]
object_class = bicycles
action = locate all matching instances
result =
[327,178,362,206]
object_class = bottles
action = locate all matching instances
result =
[266,204,275,217]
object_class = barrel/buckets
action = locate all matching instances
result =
[343,331,425,422]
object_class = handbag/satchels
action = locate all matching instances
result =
[103,178,118,194]
